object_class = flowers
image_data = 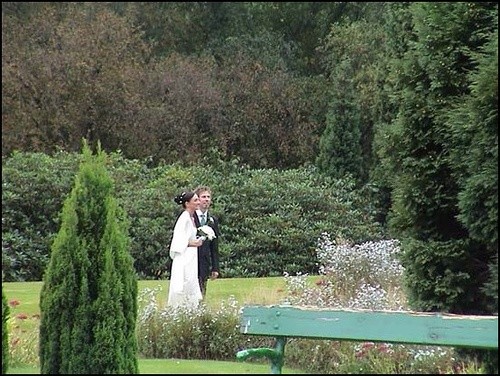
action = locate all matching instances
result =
[207,217,214,225]
[195,225,217,242]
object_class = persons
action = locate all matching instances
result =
[192,186,220,299]
[167,190,206,312]
